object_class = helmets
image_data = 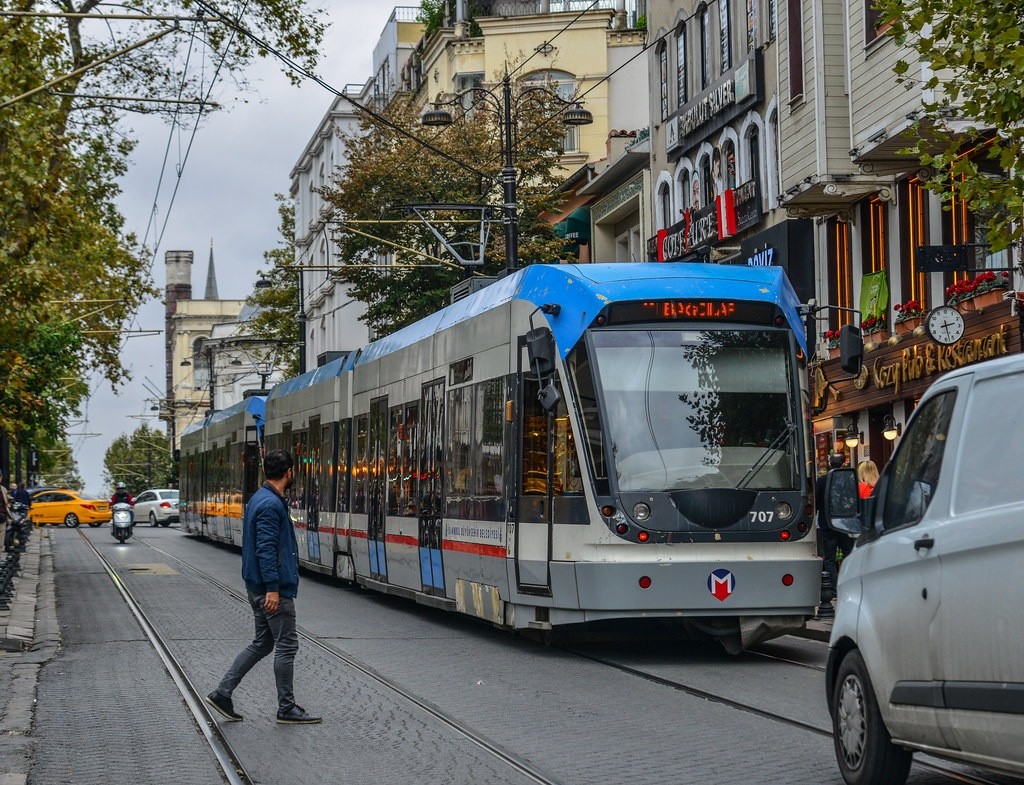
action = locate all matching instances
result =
[116,482,126,489]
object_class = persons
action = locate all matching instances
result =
[814,453,880,601]
[0,471,31,561]
[109,482,134,529]
[205,449,322,723]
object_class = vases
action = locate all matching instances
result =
[954,296,974,314]
[829,346,842,359]
[894,316,926,335]
[863,329,890,344]
[972,288,1008,310]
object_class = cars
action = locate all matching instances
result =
[23,487,112,528]
[133,488,181,529]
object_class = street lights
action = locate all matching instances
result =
[179,345,242,410]
[149,398,199,483]
[423,60,595,271]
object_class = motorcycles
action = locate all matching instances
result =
[9,501,33,552]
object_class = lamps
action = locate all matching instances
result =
[880,415,901,440]
[845,423,864,447]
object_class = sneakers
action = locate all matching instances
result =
[206,690,243,721]
[276,704,322,724]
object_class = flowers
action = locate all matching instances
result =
[945,280,972,305]
[861,314,888,335]
[894,300,925,323]
[965,270,1009,296]
[825,331,841,350]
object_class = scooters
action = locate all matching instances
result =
[109,503,136,544]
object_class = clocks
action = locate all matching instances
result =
[924,305,964,346]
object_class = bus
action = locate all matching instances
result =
[182,263,865,650]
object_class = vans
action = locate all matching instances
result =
[825,354,1024,784]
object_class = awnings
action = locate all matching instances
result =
[556,206,591,239]
